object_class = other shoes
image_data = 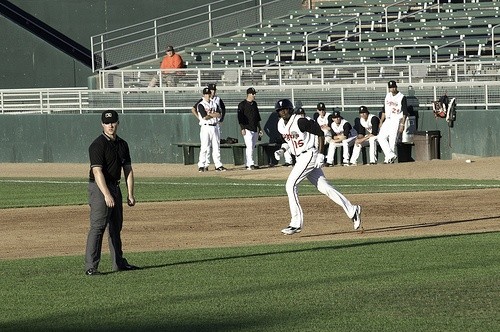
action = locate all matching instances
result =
[268,161,278,167]
[121,263,135,270]
[281,163,293,167]
[87,267,100,275]
[215,166,226,172]
[326,154,397,166]
[246,165,259,170]
[198,167,204,173]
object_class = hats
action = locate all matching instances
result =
[208,84,216,90]
[166,46,174,52]
[101,110,119,123]
[317,102,326,109]
[331,111,341,118]
[247,88,258,93]
[203,88,211,94]
[387,80,398,89]
[358,106,368,113]
[295,108,305,114]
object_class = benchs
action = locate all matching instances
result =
[169,142,416,166]
[130,0,500,71]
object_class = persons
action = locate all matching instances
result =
[376,81,408,163]
[149,46,185,92]
[348,106,382,166]
[316,102,332,144]
[283,108,311,166]
[192,84,226,171]
[323,111,356,166]
[237,88,263,169]
[274,99,362,235]
[85,110,139,274]
[197,88,226,172]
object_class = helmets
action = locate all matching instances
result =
[275,99,293,111]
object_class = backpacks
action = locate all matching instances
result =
[432,100,447,118]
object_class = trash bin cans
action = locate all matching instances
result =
[413,130,440,161]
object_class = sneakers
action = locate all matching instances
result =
[352,203,363,230]
[282,225,302,236]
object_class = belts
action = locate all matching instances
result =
[297,146,316,157]
[204,123,217,126]
[89,178,120,185]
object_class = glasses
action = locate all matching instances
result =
[252,92,256,95]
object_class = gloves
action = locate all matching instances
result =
[274,149,285,161]
[314,153,325,168]
